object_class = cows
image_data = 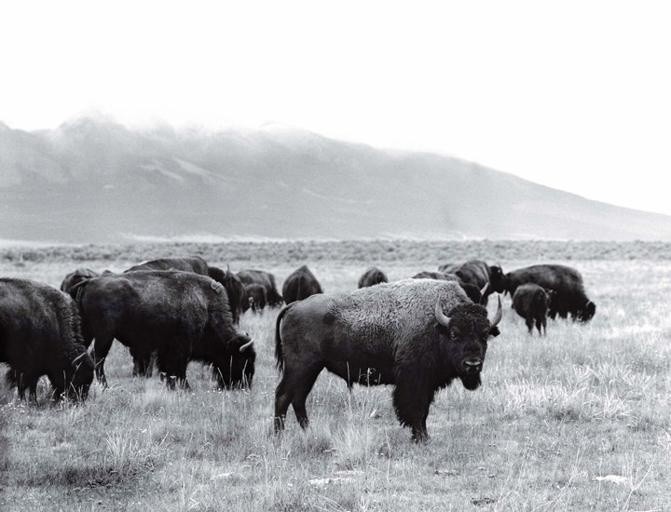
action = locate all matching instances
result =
[55,254,283,324]
[68,269,256,391]
[283,265,322,305]
[0,277,106,406]
[411,256,597,337]
[274,277,502,445]
[358,267,388,287]
